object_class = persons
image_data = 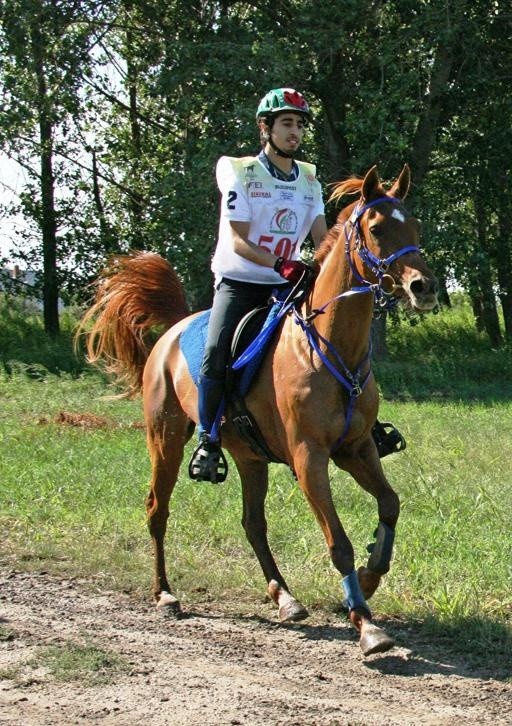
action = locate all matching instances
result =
[187,87,394,484]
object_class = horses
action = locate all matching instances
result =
[72,163,439,656]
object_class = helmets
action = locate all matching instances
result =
[255,88,311,120]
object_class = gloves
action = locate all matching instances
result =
[273,256,308,282]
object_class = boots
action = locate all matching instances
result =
[198,372,221,465]
